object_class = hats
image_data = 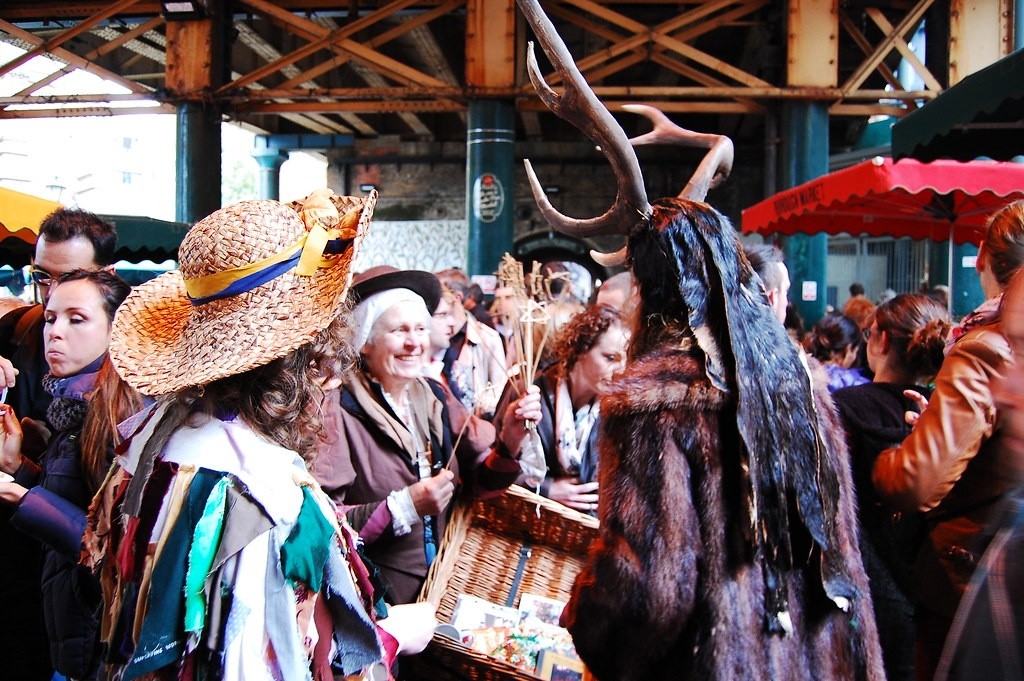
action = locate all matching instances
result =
[108,188,377,395]
[534,260,592,305]
[344,265,442,316]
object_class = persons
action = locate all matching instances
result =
[429,286,461,403]
[784,303,813,352]
[741,243,792,326]
[842,281,876,330]
[830,292,955,590]
[435,269,507,419]
[0,270,156,681]
[492,303,631,517]
[308,266,542,606]
[462,284,496,329]
[0,209,119,418]
[485,282,518,356]
[871,199,1024,681]
[558,197,885,681]
[597,271,637,312]
[809,311,870,392]
[75,188,439,681]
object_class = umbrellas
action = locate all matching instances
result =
[0,187,195,270]
[741,154,1024,327]
[891,50,1024,165]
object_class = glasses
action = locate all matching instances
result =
[863,328,882,343]
[30,266,77,287]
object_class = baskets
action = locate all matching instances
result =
[417,482,602,681]
